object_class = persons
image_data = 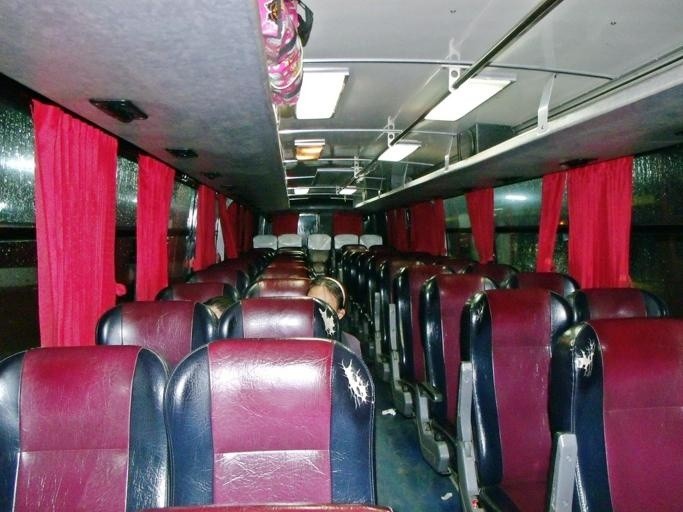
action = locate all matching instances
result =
[202,295,237,319]
[306,275,363,360]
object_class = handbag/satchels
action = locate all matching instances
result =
[258,1,313,109]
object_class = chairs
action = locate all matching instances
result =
[1,233,683,512]
